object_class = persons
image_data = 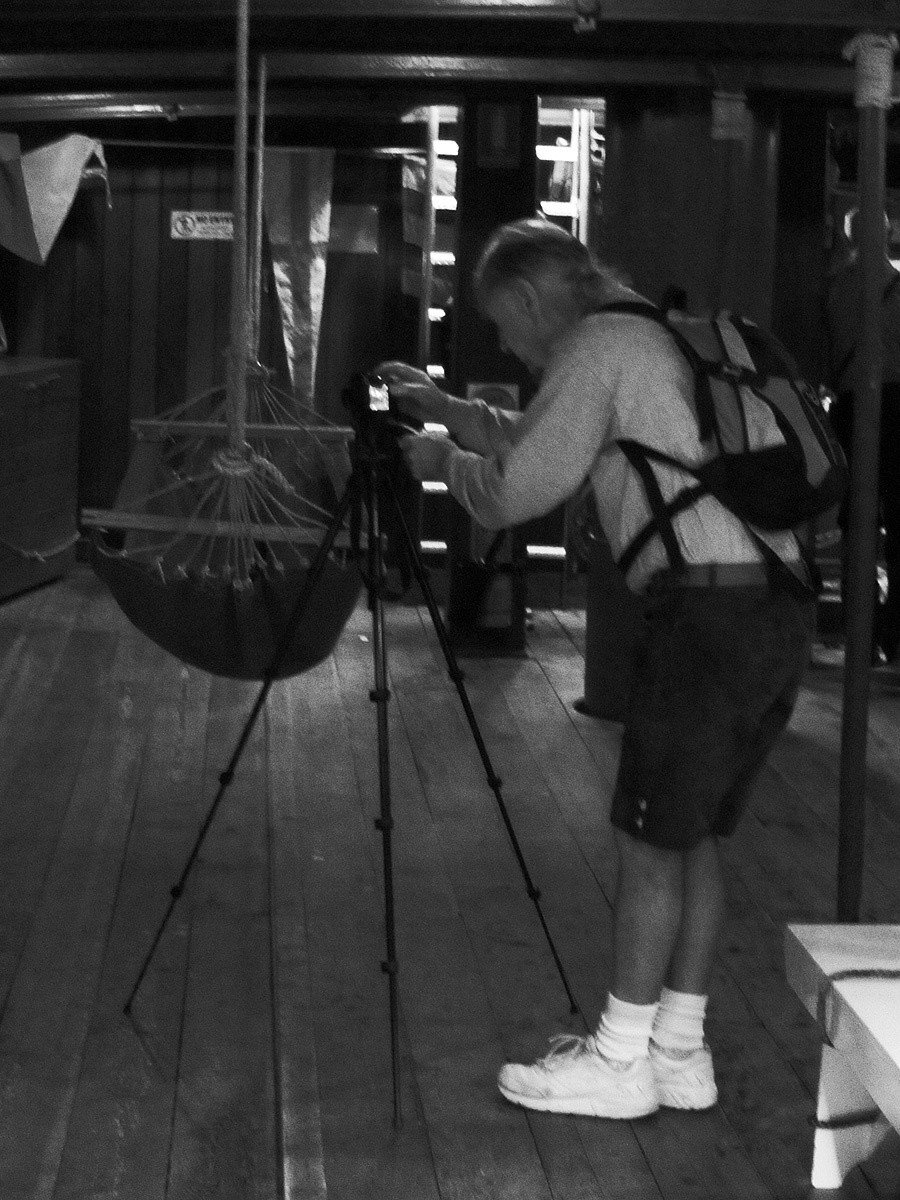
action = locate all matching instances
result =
[365,220,814,1121]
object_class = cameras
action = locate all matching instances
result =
[342,374,399,418]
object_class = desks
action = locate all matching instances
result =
[785,923,900,1190]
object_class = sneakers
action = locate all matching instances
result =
[648,1037,718,1110]
[497,1028,660,1118]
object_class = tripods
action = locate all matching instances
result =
[123,417,582,1133]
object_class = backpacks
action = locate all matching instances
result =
[590,297,853,544]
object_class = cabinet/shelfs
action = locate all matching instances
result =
[0,358,81,599]
